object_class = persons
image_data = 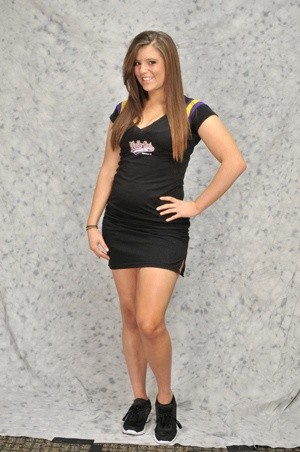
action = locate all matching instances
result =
[87,31,246,445]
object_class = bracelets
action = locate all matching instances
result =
[86,225,97,230]
[191,201,199,210]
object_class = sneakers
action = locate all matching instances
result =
[122,397,152,435]
[154,392,181,444]
